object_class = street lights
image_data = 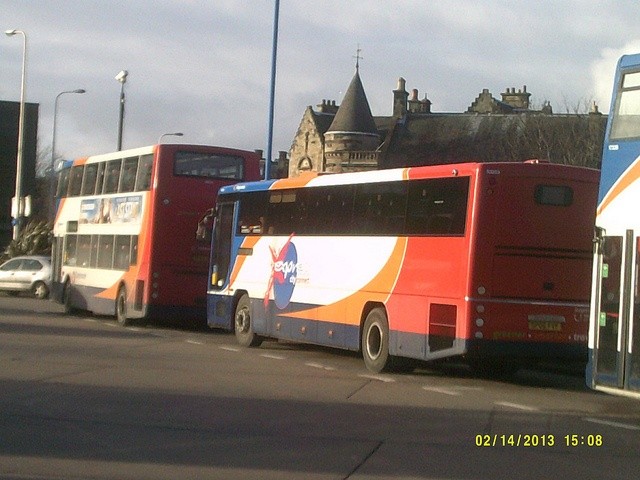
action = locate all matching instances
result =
[48,89,87,223]
[3,28,27,242]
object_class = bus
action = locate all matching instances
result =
[196,158,602,375]
[585,53,640,400]
[44,133,262,326]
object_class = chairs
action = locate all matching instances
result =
[275,214,453,234]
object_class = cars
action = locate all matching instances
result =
[0,255,52,299]
